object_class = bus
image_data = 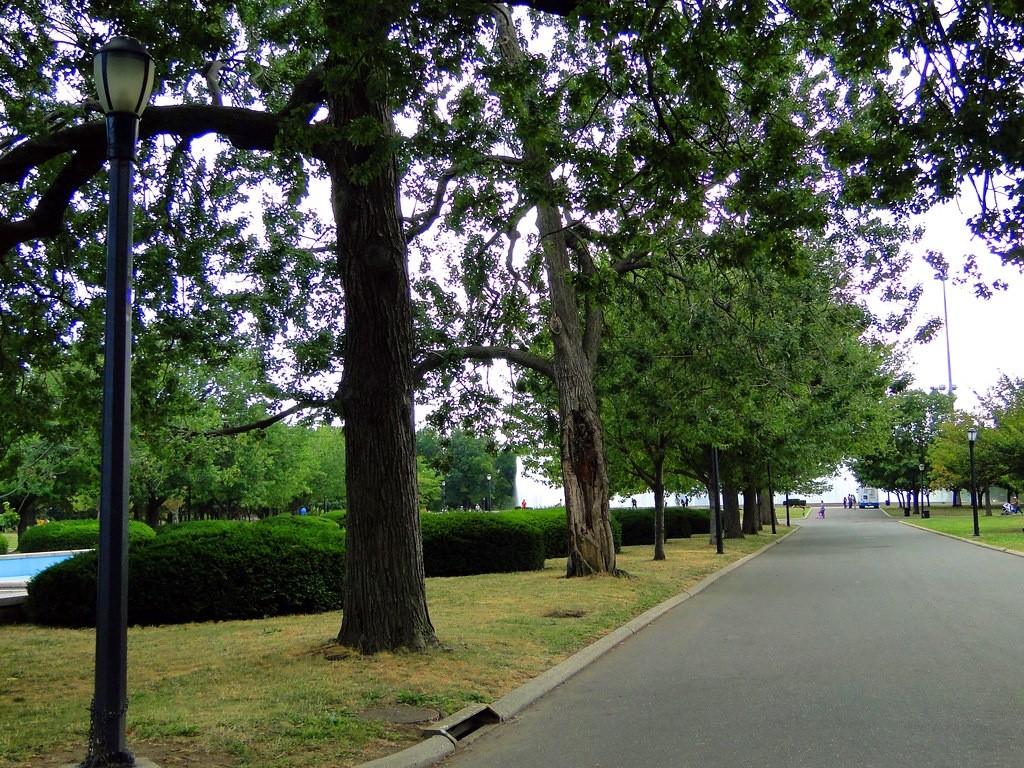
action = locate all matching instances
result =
[856,485,880,509]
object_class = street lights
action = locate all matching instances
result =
[442,480,446,514]
[967,428,980,536]
[919,463,925,518]
[79,34,158,768]
[707,402,726,553]
[486,474,492,513]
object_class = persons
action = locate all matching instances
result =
[675,495,693,507]
[664,495,667,507]
[631,498,638,510]
[522,499,526,509]
[560,498,563,506]
[300,506,306,516]
[843,494,856,510]
[818,500,825,519]
[475,503,481,512]
[1011,497,1023,514]
[802,507,806,517]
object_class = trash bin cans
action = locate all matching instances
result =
[922,510,929,518]
[886,500,890,505]
[904,508,910,517]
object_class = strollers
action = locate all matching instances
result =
[1001,503,1017,516]
